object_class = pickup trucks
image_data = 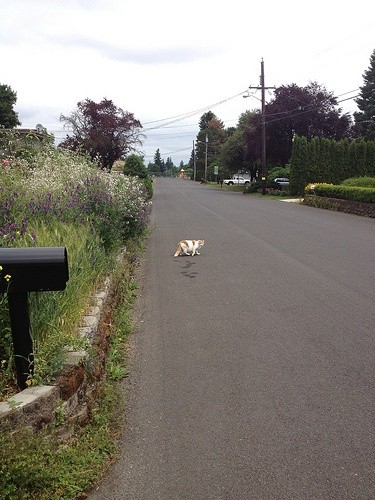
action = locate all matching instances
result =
[223,176,250,185]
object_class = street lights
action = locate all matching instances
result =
[243,94,268,176]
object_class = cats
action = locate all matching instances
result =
[173,246,205,257]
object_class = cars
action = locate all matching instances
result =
[273,177,290,187]
